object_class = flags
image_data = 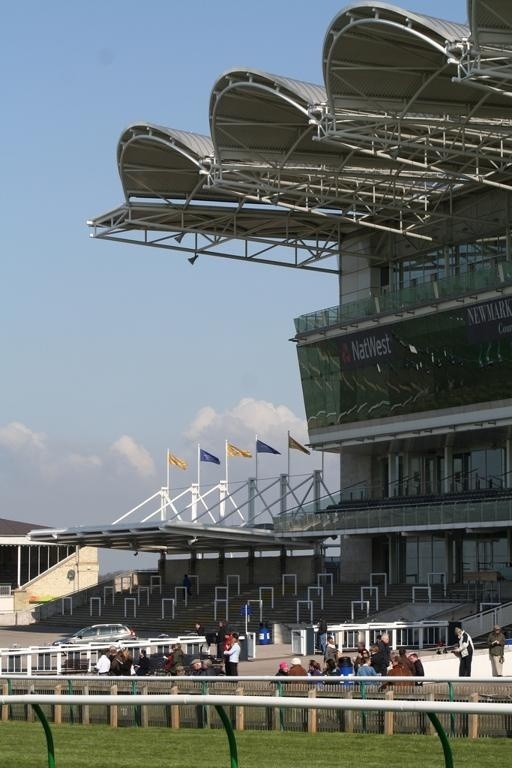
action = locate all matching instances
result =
[200,448,220,465]
[289,435,310,455]
[169,451,187,471]
[257,440,282,455]
[227,442,252,458]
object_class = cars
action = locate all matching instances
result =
[52,623,137,656]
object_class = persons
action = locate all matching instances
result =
[183,574,193,600]
[451,627,474,678]
[94,620,242,676]
[270,619,424,695]
[486,625,507,677]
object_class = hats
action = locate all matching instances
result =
[203,659,212,666]
[291,658,300,664]
[455,628,461,634]
[176,666,183,671]
[280,663,288,669]
[191,659,201,664]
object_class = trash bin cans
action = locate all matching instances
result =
[337,657,353,688]
